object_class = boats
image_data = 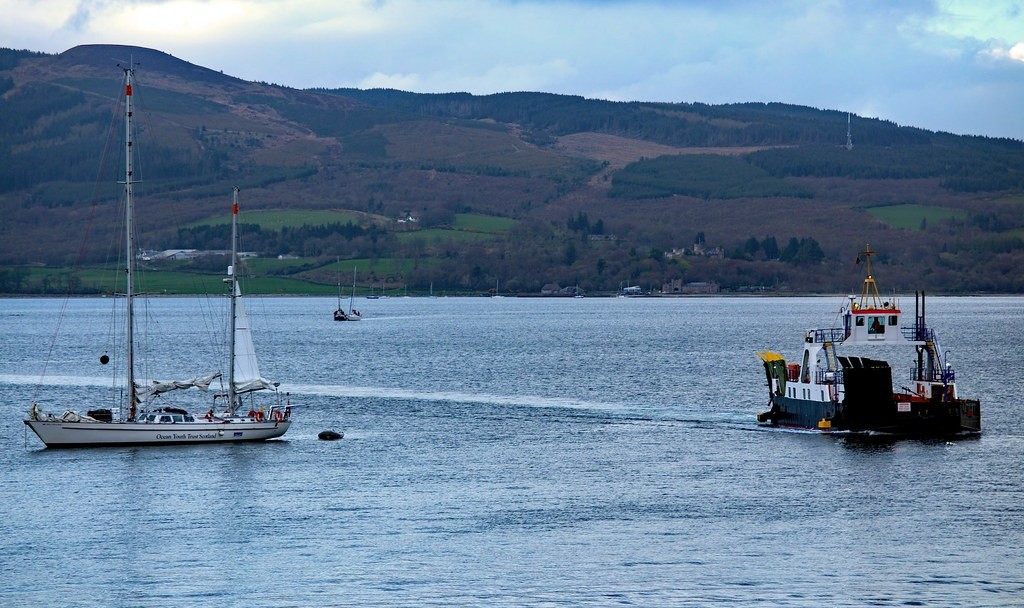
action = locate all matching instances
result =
[755,241,982,439]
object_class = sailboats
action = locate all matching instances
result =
[331,254,364,323]
[22,60,303,450]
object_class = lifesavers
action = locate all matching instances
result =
[248,410,256,417]
[274,411,282,421]
[256,411,262,421]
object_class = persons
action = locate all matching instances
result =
[205,409,213,422]
[870,317,880,334]
[274,409,283,422]
[857,318,863,326]
